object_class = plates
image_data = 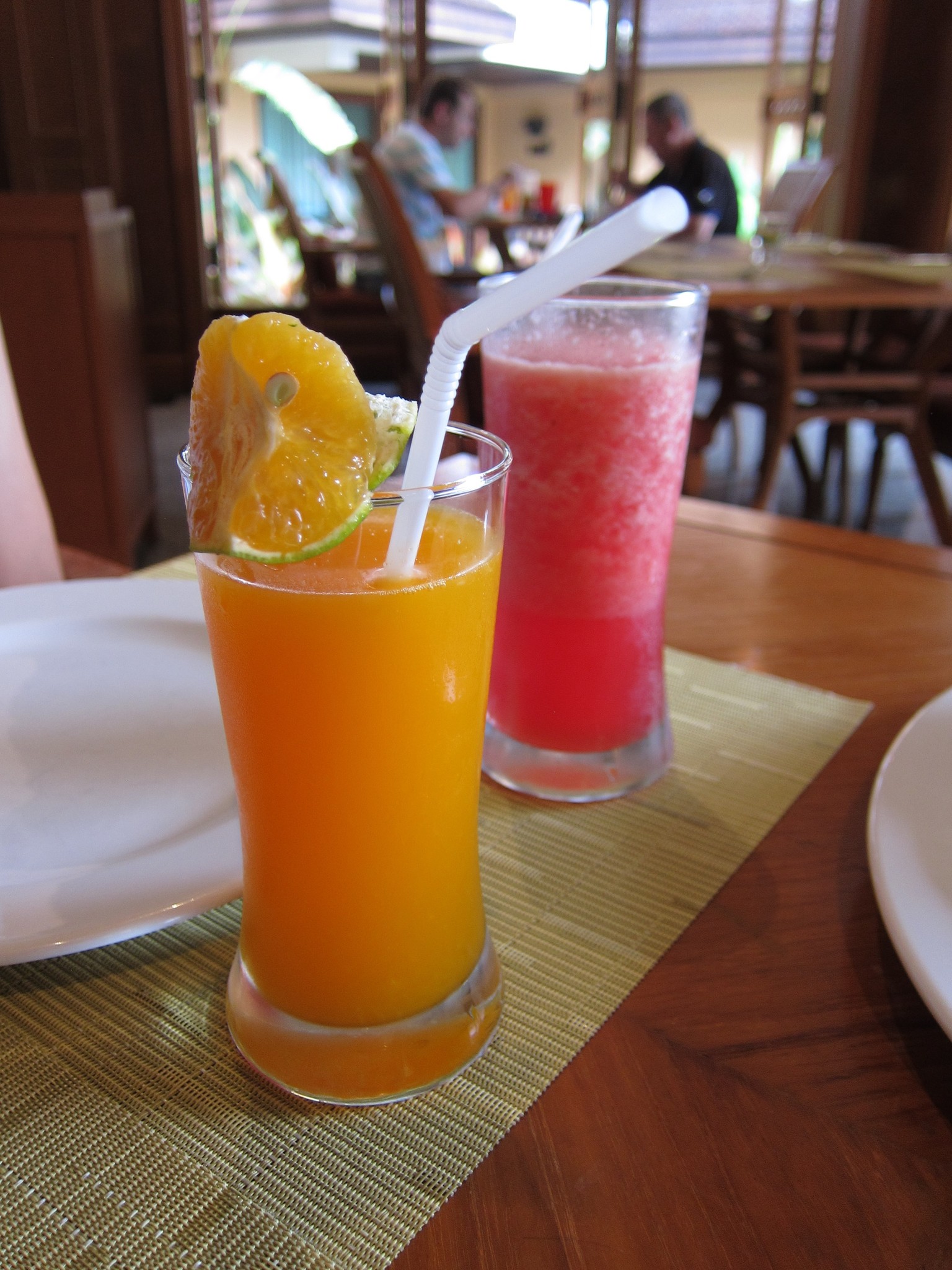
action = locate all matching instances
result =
[867,679,951,1048]
[1,576,243,974]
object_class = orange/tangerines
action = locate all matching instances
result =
[181,310,377,557]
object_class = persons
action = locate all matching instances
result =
[604,94,738,243]
[370,72,541,280]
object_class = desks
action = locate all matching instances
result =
[0,452,952,1270]
[644,252,952,542]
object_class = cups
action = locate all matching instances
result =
[478,269,717,804]
[175,421,512,1109]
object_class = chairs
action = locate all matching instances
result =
[255,141,951,549]
